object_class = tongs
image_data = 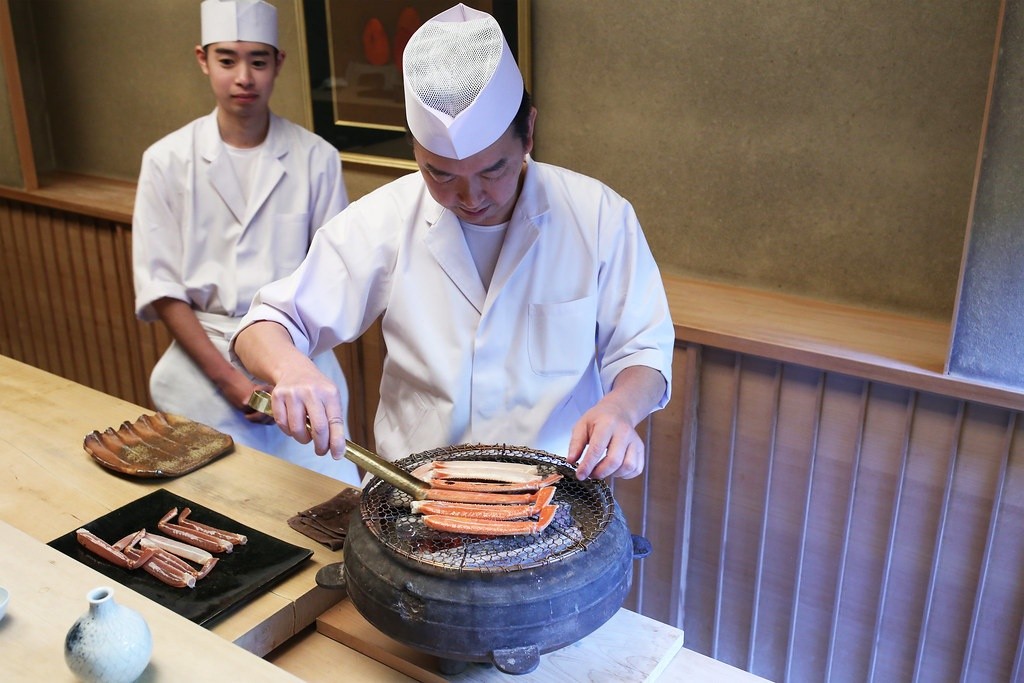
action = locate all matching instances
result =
[248,389,432,500]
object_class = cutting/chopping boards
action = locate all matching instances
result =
[316,594,684,683]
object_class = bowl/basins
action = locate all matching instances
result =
[0,587,10,621]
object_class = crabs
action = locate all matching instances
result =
[410,459,564,535]
[76,507,249,589]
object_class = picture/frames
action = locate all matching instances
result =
[294,1,532,172]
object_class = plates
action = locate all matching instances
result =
[46,488,314,627]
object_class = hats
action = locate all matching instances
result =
[403,2,528,160]
[201,0,278,50]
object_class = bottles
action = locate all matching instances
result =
[64,586,152,683]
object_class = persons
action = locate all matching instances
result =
[132,0,362,489]
[229,4,677,486]
